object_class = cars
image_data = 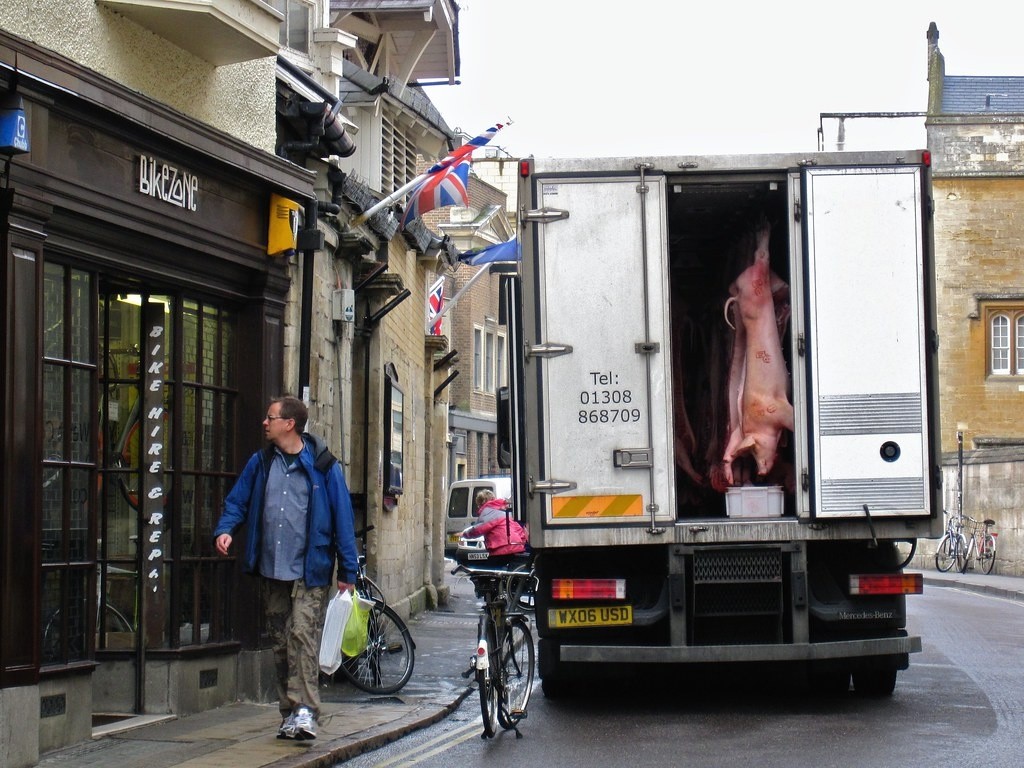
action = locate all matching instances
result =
[454,523,494,567]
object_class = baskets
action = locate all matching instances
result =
[970,521,985,536]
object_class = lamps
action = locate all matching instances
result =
[0,77,31,153]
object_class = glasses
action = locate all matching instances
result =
[266,415,292,424]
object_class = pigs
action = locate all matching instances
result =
[668,228,794,494]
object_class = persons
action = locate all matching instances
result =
[211,395,358,741]
[453,489,527,590]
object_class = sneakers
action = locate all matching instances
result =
[293,706,317,740]
[277,711,296,739]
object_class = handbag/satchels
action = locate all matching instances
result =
[341,588,370,657]
[319,587,361,675]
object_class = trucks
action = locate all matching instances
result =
[495,148,945,698]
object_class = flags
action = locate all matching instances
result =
[400,123,504,232]
[454,237,522,267]
[429,284,444,335]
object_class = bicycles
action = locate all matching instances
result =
[339,525,416,695]
[960,515,1000,575]
[935,509,971,573]
[451,563,539,739]
[447,537,537,613]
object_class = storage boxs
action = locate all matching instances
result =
[725,487,785,518]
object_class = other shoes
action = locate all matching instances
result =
[475,601,487,613]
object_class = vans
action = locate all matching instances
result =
[442,474,513,552]
